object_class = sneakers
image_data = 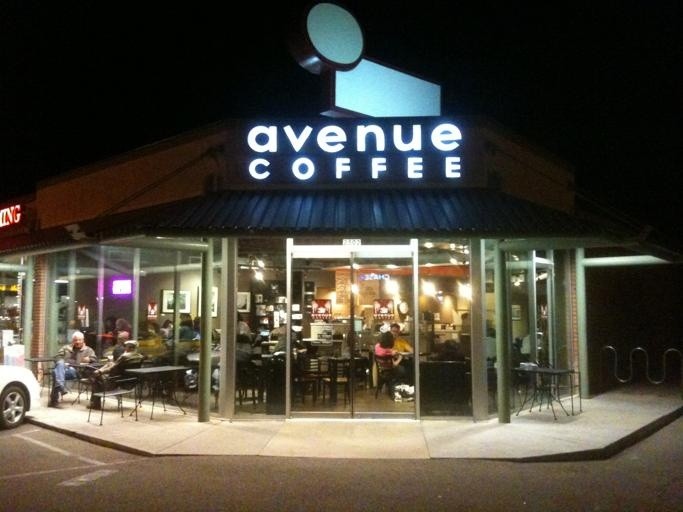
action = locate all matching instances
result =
[52,401,58,406]
[58,385,67,395]
[79,379,93,384]
[87,403,100,410]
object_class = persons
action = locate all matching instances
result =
[374,331,409,400]
[389,323,414,385]
[85,313,214,409]
[237,310,252,352]
[47,331,98,408]
[272,331,305,392]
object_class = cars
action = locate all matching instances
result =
[0,365,41,430]
[181,341,219,393]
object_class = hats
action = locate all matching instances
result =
[124,340,137,347]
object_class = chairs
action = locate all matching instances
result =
[24,328,584,427]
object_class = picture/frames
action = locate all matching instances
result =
[161,289,191,314]
[198,284,218,318]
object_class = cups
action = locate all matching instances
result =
[108,355,114,363]
